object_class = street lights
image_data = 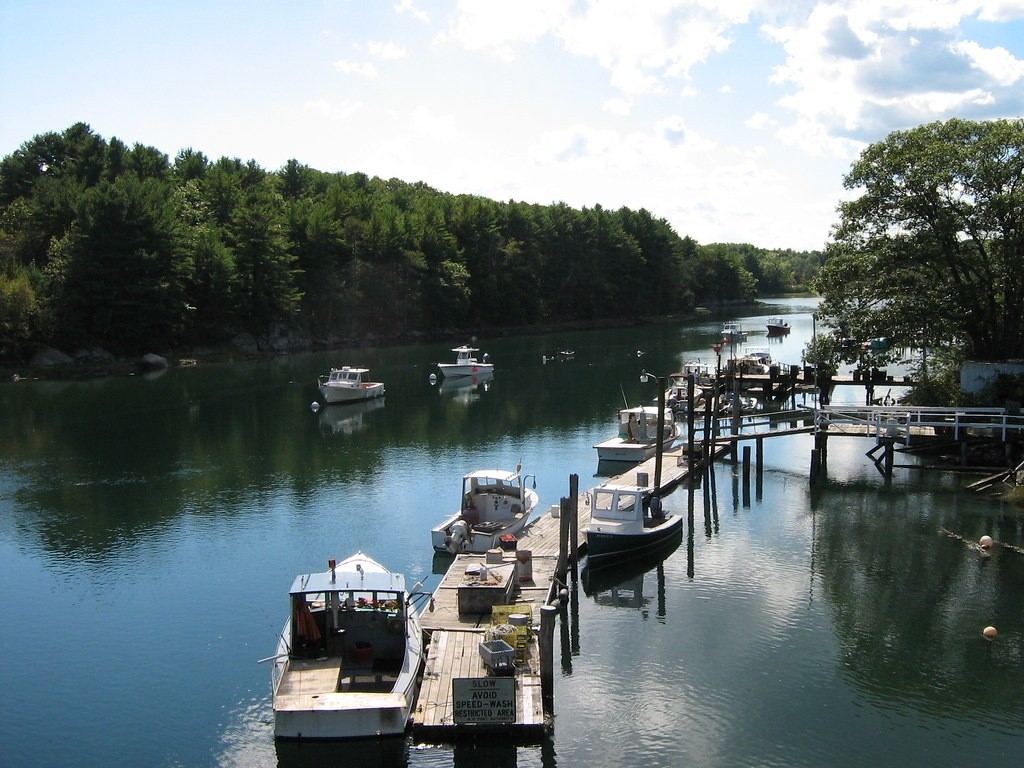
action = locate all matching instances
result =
[639,368,665,488]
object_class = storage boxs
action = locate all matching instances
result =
[499,535,517,551]
[483,605,532,666]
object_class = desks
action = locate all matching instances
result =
[457,563,516,624]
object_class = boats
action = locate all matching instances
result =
[720,321,747,341]
[727,397,758,413]
[695,308,712,315]
[593,383,682,462]
[179,359,198,368]
[317,365,385,403]
[270,550,423,738]
[651,357,712,412]
[579,469,684,572]
[437,345,494,377]
[733,348,773,376]
[767,318,790,336]
[431,459,538,553]
